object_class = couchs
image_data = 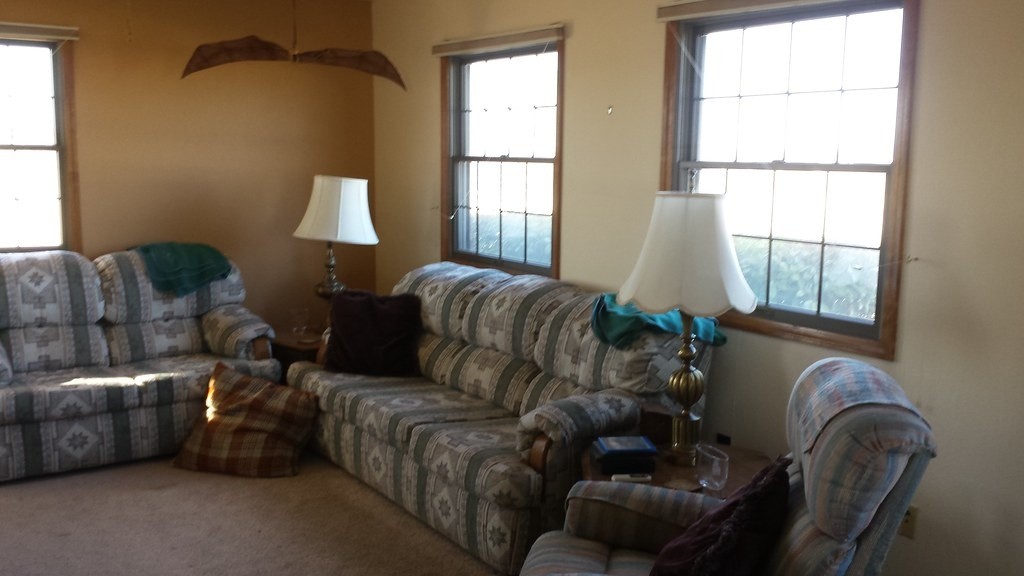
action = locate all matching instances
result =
[518,356,936,576]
[285,259,715,575]
[0,250,281,485]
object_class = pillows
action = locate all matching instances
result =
[321,289,424,378]
[172,360,320,480]
[650,453,793,576]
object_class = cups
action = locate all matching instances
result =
[693,444,729,491]
[292,323,318,343]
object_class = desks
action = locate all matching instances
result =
[584,437,771,498]
[273,330,322,363]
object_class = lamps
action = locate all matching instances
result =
[292,175,379,339]
[615,169,758,465]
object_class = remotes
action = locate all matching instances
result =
[612,473,652,482]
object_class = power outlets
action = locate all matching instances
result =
[715,433,732,446]
[899,506,918,539]
